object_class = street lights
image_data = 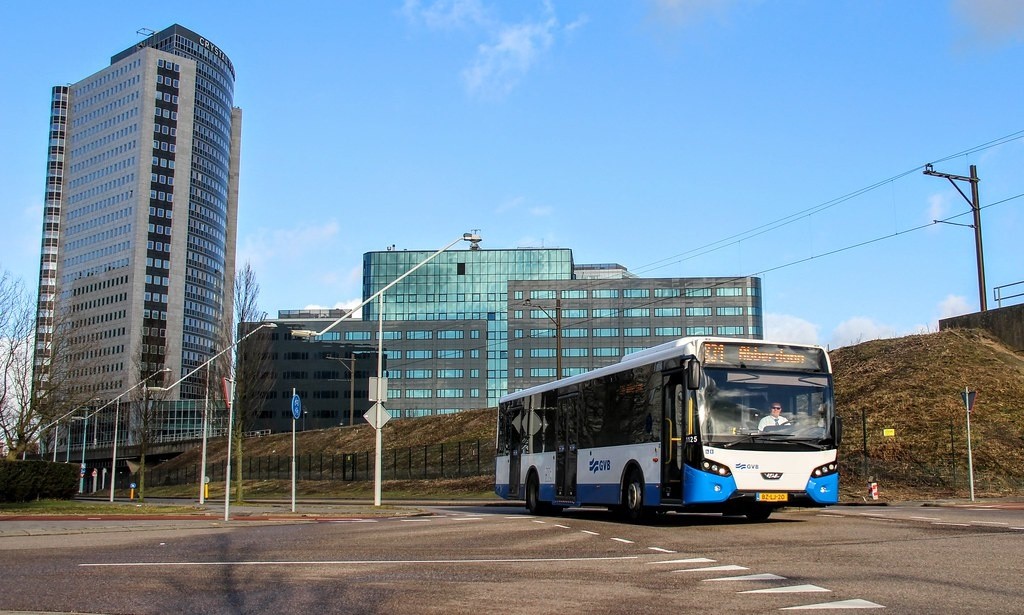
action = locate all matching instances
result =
[35,398,100,462]
[69,367,171,502]
[147,324,280,504]
[289,233,482,506]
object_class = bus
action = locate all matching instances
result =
[492,336,844,523]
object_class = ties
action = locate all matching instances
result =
[775,419,779,426]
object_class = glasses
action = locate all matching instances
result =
[772,407,780,410]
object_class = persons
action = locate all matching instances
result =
[758,399,791,434]
